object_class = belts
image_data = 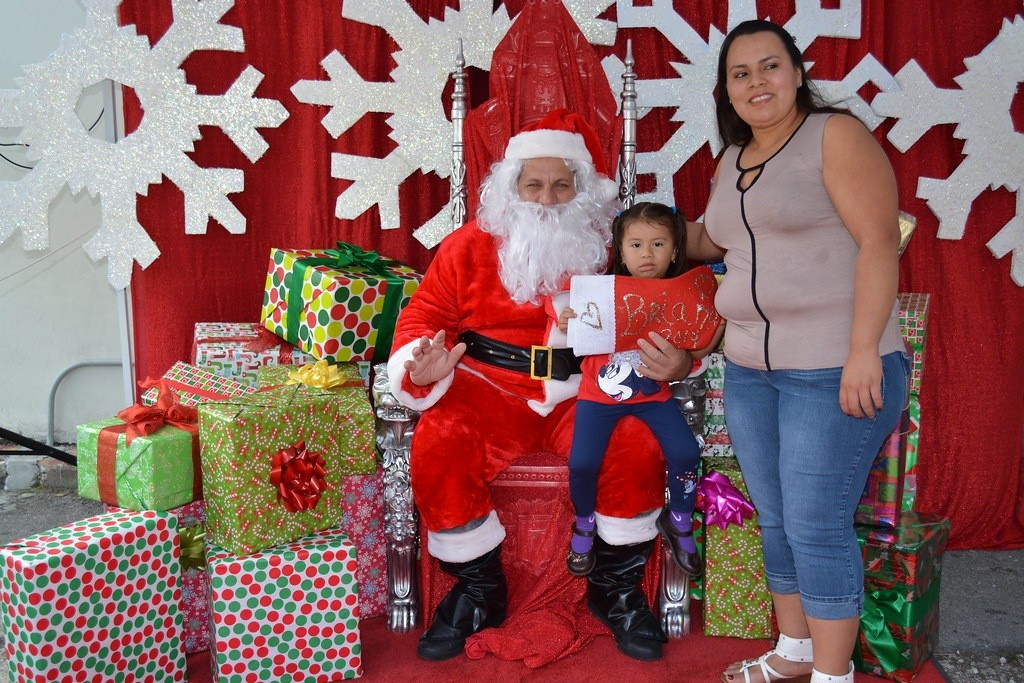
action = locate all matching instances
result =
[456,329,586,381]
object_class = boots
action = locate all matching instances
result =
[586,508,669,662]
[417,510,506,660]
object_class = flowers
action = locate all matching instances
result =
[325,240,385,276]
[269,441,327,515]
[113,378,199,434]
[695,464,758,533]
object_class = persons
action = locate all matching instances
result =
[683,15,910,683]
[386,108,696,661]
[557,201,727,577]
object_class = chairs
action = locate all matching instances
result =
[371,36,701,638]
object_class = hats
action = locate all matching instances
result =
[504,108,619,204]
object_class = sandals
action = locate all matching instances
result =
[810,660,855,683]
[721,634,813,683]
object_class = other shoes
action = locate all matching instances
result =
[655,508,705,577]
[567,519,597,574]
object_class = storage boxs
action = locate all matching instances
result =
[0,245,408,683]
[689,257,950,673]
